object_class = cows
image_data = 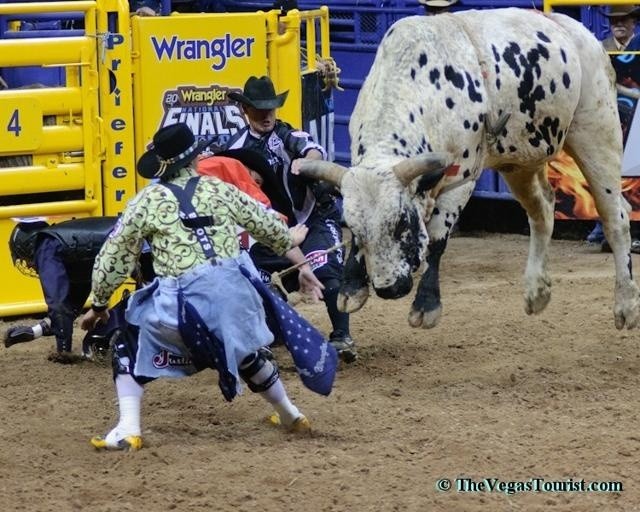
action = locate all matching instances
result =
[297,7,640,331]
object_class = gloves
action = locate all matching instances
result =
[4,319,50,348]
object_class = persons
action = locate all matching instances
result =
[8,75,357,453]
[135,1,162,16]
[584,3,640,254]
[419,0,456,17]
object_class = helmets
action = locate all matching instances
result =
[9,220,48,277]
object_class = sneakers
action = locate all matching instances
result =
[270,408,311,434]
[92,433,142,452]
[327,330,358,362]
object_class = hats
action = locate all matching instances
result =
[214,148,280,202]
[228,76,288,109]
[601,5,639,17]
[419,0,457,7]
[137,123,217,177]
